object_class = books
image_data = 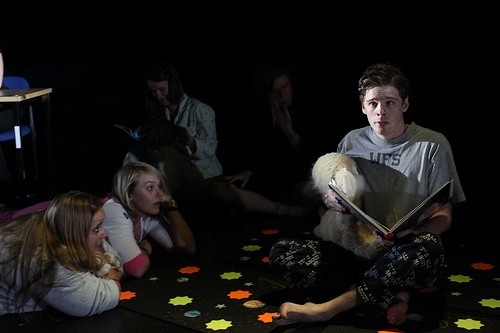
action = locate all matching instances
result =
[112,113,181,145]
[327,179,454,250]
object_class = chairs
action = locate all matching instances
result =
[2,76,36,138]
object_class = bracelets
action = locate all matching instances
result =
[165,206,179,212]
[141,247,149,255]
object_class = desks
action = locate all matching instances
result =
[0,87,53,139]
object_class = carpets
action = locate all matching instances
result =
[118,216,500,333]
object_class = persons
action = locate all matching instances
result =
[135,58,324,218]
[223,67,320,213]
[0,190,125,327]
[0,161,197,278]
[268,61,467,327]
